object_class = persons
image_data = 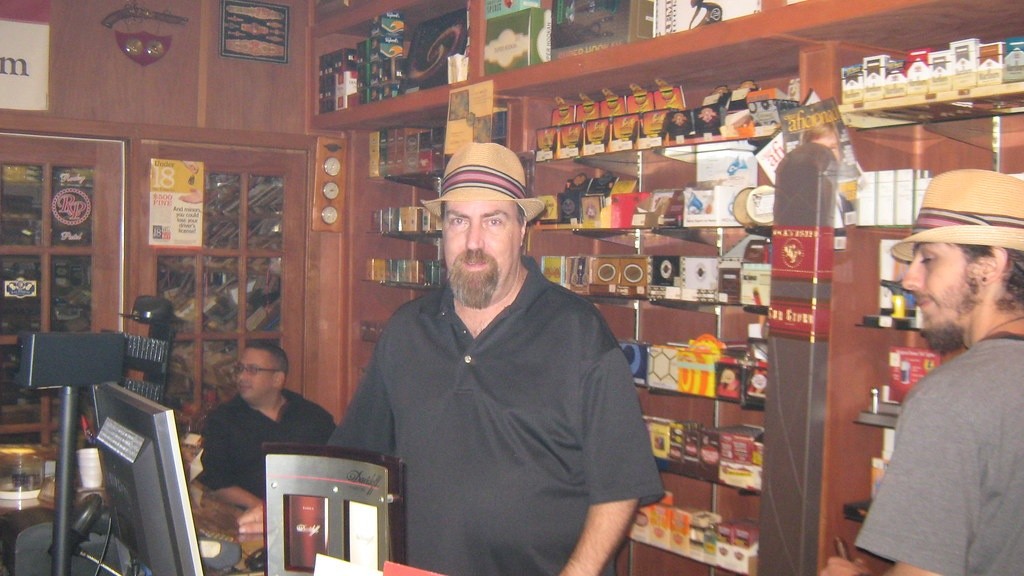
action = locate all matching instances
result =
[820,168,1024,576]
[237,142,667,576]
[800,120,841,167]
[201,341,337,508]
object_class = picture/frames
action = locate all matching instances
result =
[398,9,468,96]
[219,0,289,64]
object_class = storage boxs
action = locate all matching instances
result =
[370,61,383,84]
[371,11,404,36]
[333,47,357,72]
[384,81,400,100]
[320,53,336,75]
[369,36,403,63]
[336,70,357,110]
[319,73,335,93]
[319,93,336,114]
[383,57,406,80]
[484,0,762,78]
[356,40,371,63]
[357,64,369,91]
[359,88,369,103]
[369,85,383,104]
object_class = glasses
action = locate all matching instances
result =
[234,365,279,375]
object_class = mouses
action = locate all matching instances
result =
[244,547,265,572]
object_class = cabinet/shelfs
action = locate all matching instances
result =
[534,123,776,576]
[842,110,1023,526]
[361,168,445,340]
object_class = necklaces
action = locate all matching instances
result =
[983,316,1024,336]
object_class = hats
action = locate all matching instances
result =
[890,168,1024,261]
[420,142,545,223]
[117,295,186,324]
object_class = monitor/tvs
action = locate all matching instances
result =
[92,382,205,576]
